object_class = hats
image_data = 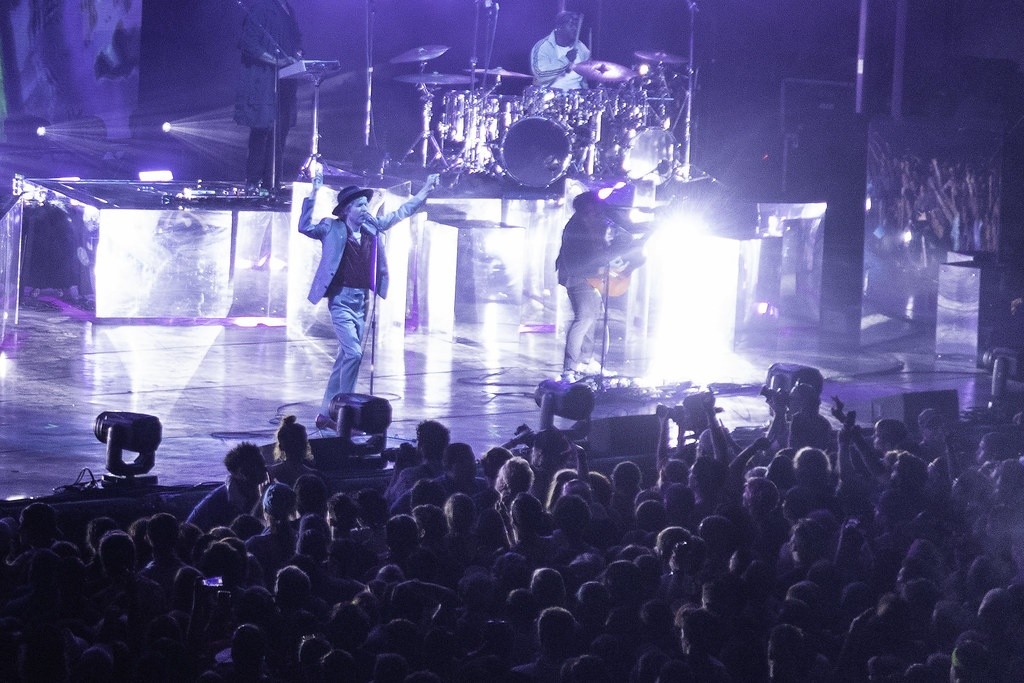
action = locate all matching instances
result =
[332,185,374,216]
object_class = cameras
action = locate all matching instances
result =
[655,404,684,425]
[759,384,790,407]
[514,424,537,449]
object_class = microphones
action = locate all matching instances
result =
[366,216,385,234]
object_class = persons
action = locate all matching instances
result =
[555,190,651,378]
[298,170,440,438]
[0,381,1024,683]
[233,0,305,197]
[528,11,591,94]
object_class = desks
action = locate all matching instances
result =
[937,261,1024,369]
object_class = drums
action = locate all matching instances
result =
[499,112,574,189]
[600,125,680,188]
[444,88,521,144]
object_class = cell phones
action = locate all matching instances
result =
[203,577,225,587]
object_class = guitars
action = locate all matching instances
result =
[586,201,673,299]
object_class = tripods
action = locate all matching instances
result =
[297,78,336,182]
[673,0,725,188]
[397,62,505,189]
[571,106,614,180]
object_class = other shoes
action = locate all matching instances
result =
[574,363,596,376]
[561,370,577,384]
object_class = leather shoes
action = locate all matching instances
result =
[350,428,367,437]
[316,414,338,431]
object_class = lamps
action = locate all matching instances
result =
[656,390,724,440]
[766,363,824,421]
[327,392,392,470]
[533,380,596,451]
[93,411,163,486]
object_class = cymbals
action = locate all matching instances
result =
[462,67,534,78]
[633,48,687,65]
[390,44,451,62]
[392,72,481,85]
[572,60,640,81]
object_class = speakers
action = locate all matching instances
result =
[570,415,670,460]
[773,78,866,252]
[259,436,358,469]
[872,389,960,428]
